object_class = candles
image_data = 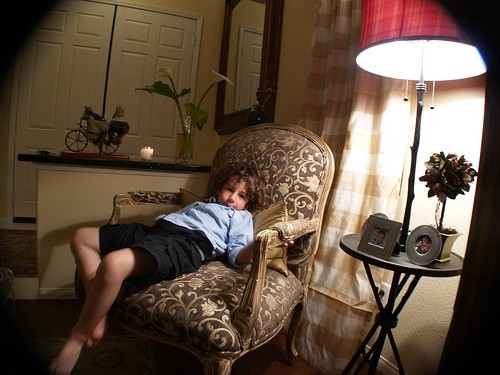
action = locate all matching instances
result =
[139,146,154,161]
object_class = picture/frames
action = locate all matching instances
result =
[357,214,404,260]
[405,225,443,266]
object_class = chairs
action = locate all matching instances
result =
[68,122,335,375]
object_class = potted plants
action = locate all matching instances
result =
[419,151,477,262]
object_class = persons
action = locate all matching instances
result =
[372,231,387,248]
[48,162,293,374]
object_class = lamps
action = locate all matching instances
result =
[355,0,490,248]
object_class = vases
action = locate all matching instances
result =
[175,115,197,164]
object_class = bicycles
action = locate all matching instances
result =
[65,116,120,155]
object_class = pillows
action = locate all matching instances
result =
[180,187,289,274]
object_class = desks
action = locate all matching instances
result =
[338,230,466,375]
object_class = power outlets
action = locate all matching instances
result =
[377,282,392,307]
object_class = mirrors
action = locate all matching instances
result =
[215,0,284,135]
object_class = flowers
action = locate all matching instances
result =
[137,69,234,160]
